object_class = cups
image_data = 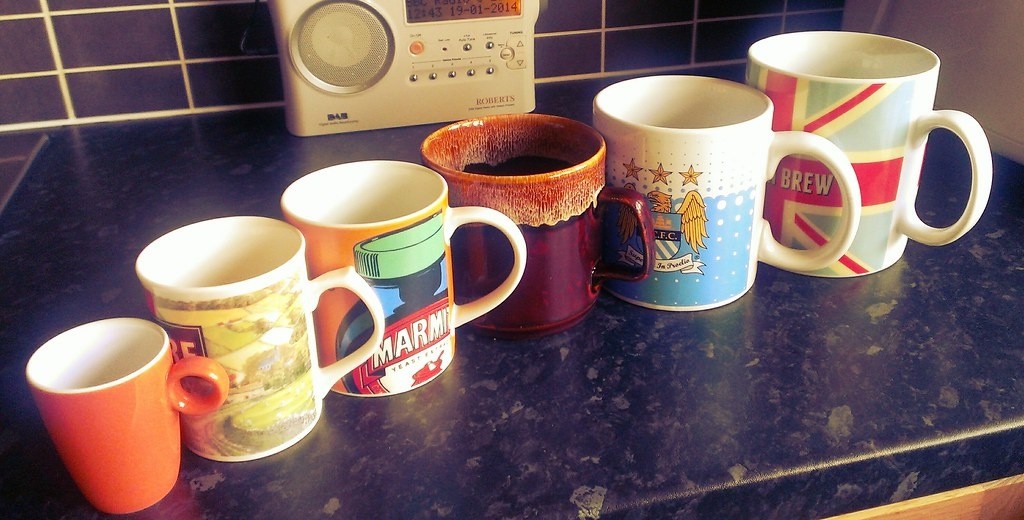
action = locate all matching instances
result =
[280,159,528,397]
[136,216,386,463]
[25,316,230,515]
[746,31,994,278]
[592,75,862,312]
[421,114,657,340]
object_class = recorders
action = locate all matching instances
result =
[268,0,540,138]
